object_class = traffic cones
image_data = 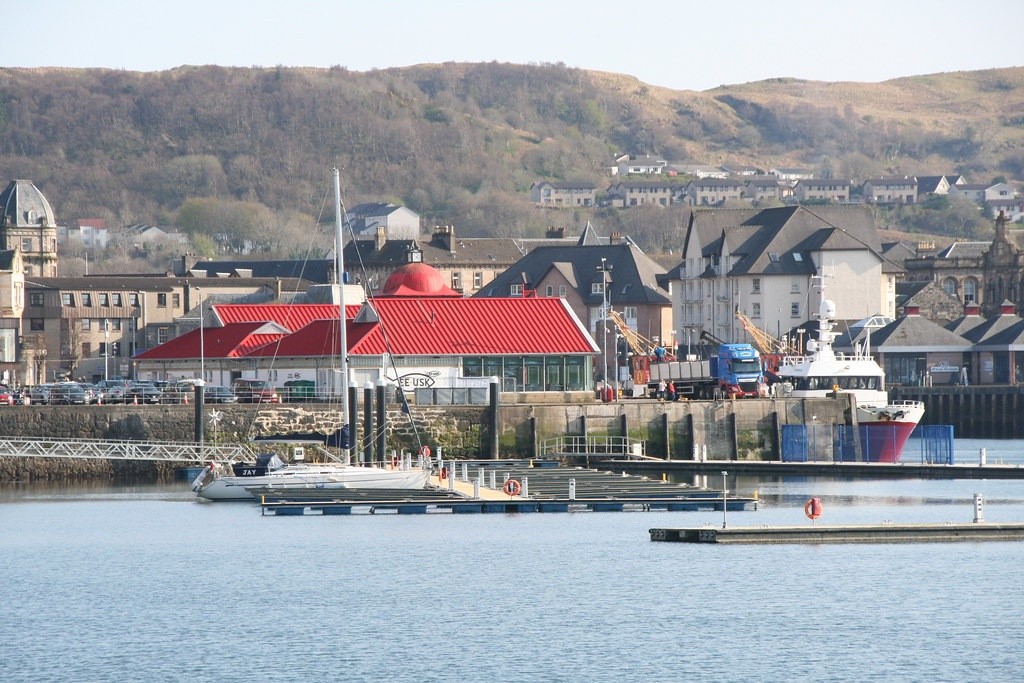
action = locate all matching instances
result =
[182,394,190,405]
[133,394,138,405]
[96,395,102,406]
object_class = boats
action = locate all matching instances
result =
[605,265,928,464]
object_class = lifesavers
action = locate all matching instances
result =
[805,499,823,519]
[419,446,431,458]
[503,479,520,495]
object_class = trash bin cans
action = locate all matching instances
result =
[284,380,315,402]
[414,388,433,405]
[433,388,452,405]
[452,388,470,405]
[469,387,486,405]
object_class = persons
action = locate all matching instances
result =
[766,379,773,400]
[755,376,761,399]
[654,346,663,364]
[658,379,667,398]
[669,380,676,400]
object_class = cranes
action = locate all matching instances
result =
[733,302,802,357]
[607,309,676,359]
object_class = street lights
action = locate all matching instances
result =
[721,470,729,530]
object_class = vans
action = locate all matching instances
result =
[231,378,280,404]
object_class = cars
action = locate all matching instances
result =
[283,381,316,401]
[0,379,196,404]
[204,385,239,403]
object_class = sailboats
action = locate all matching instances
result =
[187,165,477,503]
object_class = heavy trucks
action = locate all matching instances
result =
[647,343,765,400]
